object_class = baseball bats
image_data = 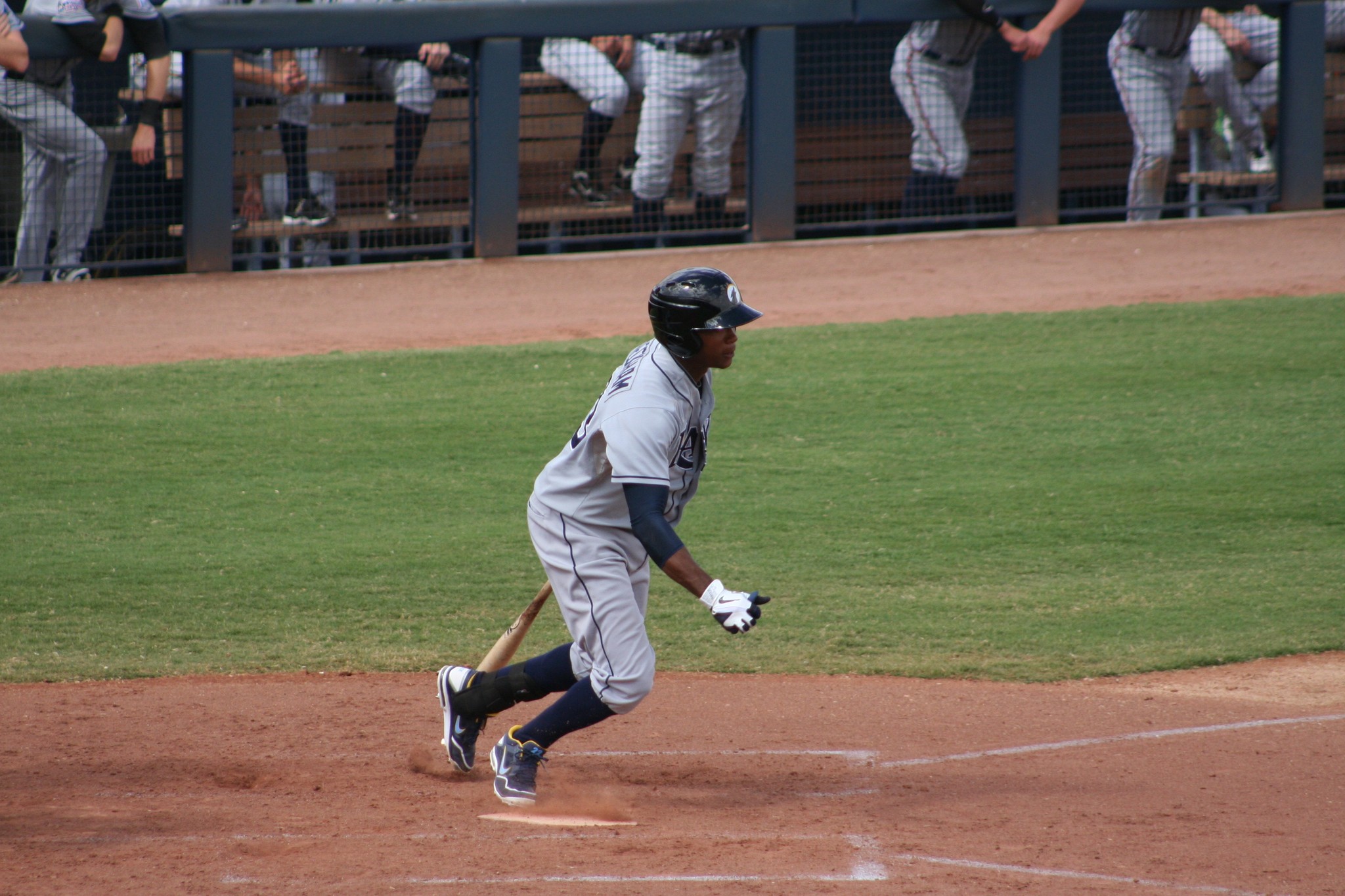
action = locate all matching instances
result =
[473,579,552,672]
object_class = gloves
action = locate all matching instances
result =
[700,579,771,635]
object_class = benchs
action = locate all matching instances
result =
[164,45,1344,275]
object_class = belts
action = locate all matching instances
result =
[643,36,735,54]
[1129,42,1191,60]
[917,48,973,66]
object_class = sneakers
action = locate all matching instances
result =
[1213,114,1237,150]
[385,198,418,221]
[567,168,613,205]
[283,197,335,226]
[436,666,496,772]
[51,266,91,282]
[490,724,549,809]
[1247,145,1274,172]
[230,216,248,232]
[610,163,633,194]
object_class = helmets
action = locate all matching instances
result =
[647,267,763,358]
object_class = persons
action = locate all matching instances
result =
[892,0,1280,224]
[541,30,748,246]
[0,0,449,284]
[437,266,771,807]
